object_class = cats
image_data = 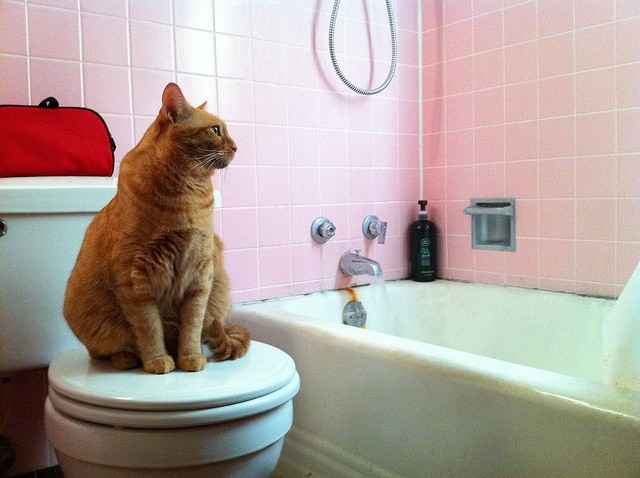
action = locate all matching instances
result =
[62,82,252,375]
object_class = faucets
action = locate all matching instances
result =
[340,249,383,280]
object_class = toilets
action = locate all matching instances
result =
[0,174,302,478]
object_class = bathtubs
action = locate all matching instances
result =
[230,278,638,478]
